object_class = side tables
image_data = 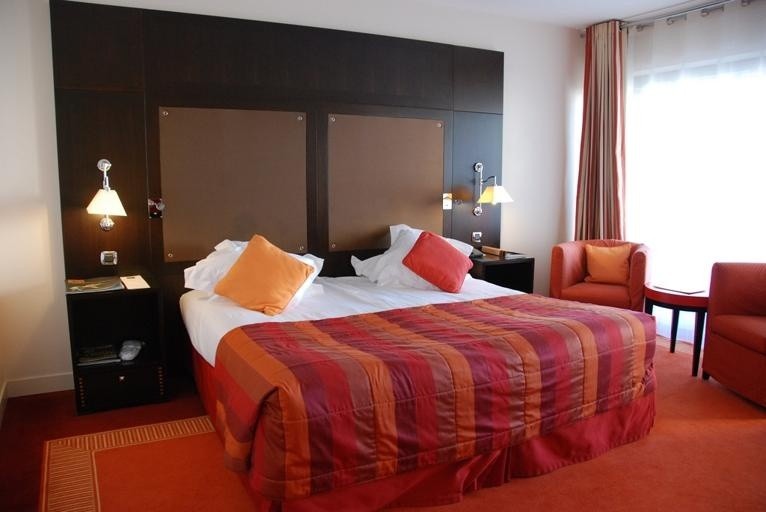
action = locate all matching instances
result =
[628,276,709,379]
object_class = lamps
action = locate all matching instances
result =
[85,158,128,231]
[473,162,509,217]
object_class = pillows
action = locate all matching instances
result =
[212,233,315,317]
[184,238,325,306]
[403,229,474,293]
[584,243,632,285]
[389,223,414,244]
[350,227,473,292]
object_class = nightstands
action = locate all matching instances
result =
[460,246,537,295]
[64,264,173,417]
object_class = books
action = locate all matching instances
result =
[79,344,117,362]
[65,275,126,295]
[119,275,151,290]
[76,357,122,367]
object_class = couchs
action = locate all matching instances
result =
[701,262,766,412]
[547,236,644,319]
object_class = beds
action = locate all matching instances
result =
[167,241,659,506]
